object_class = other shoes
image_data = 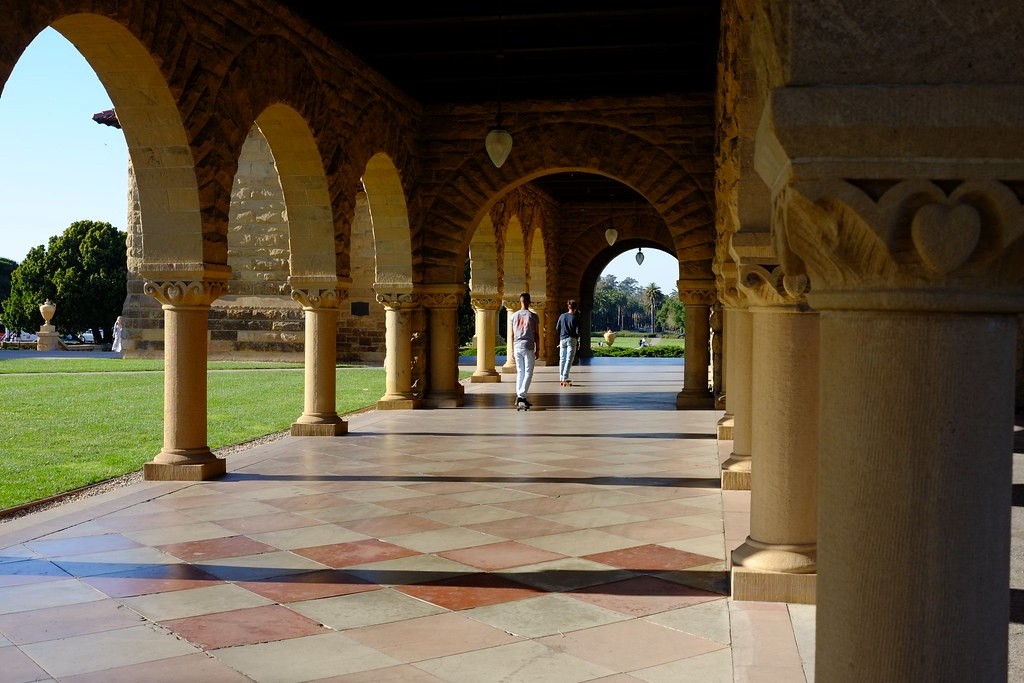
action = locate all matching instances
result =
[560,377,572,382]
[515,397,533,407]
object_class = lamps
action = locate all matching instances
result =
[604,193,618,246]
[635,245,643,264]
[484,52,513,170]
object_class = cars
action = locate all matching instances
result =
[78,329,103,344]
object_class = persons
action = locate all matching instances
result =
[112,316,122,353]
[556,300,580,382]
[510,292,540,406]
[641,335,648,348]
